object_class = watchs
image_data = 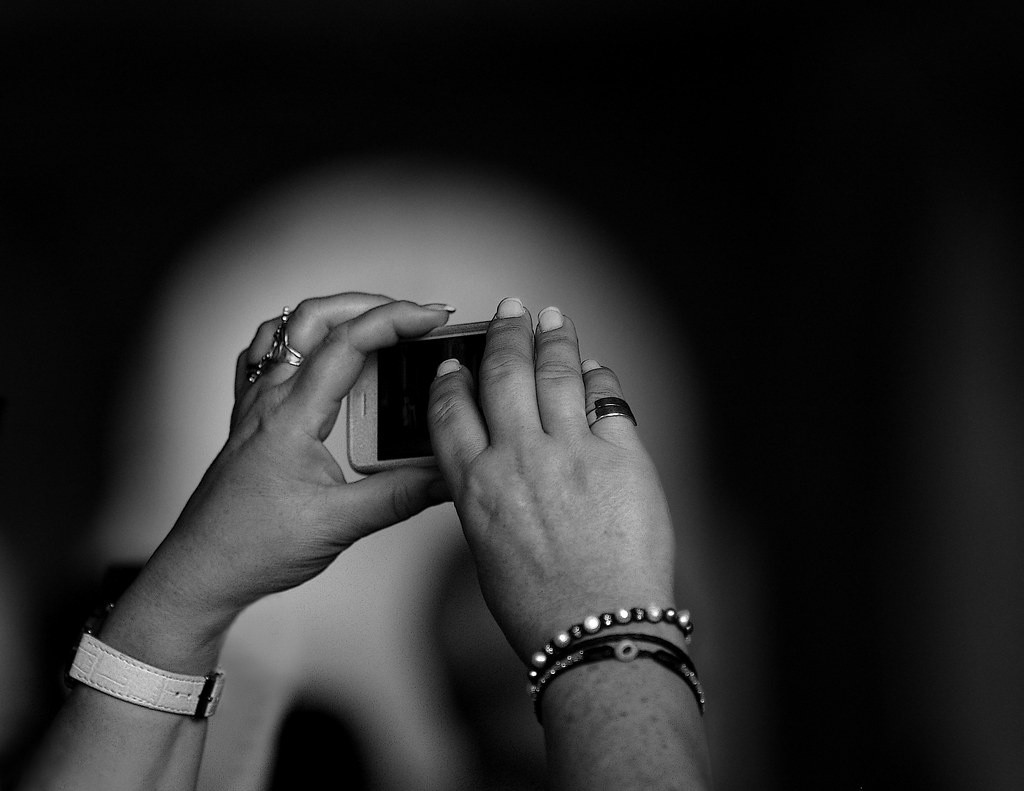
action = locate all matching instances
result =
[64,602,225,719]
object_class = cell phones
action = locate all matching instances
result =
[346,320,497,476]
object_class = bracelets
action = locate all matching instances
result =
[527,604,706,727]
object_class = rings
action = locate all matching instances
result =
[249,305,305,382]
[584,397,638,427]
[246,368,257,373]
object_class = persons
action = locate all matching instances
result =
[27,287,716,791]
